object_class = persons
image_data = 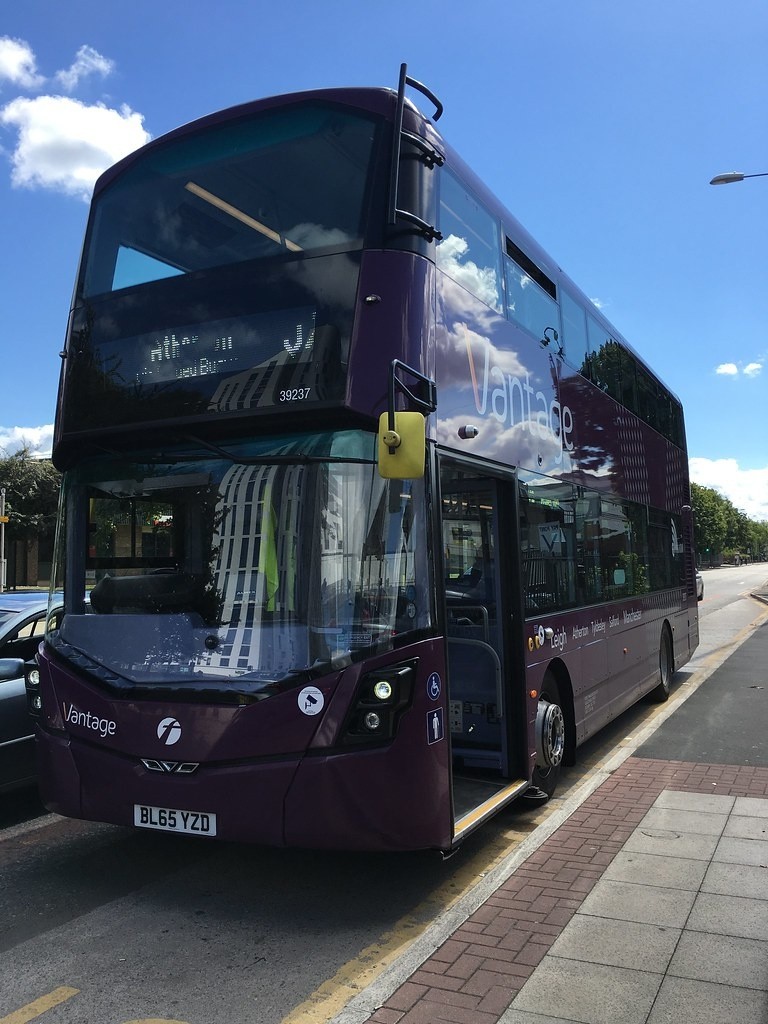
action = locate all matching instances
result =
[745,557,748,565]
[467,559,538,608]
[457,544,494,585]
[740,557,743,565]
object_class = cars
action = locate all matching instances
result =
[0,592,95,807]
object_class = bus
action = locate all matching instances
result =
[33,61,701,862]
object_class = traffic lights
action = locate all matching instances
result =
[706,546,710,551]
